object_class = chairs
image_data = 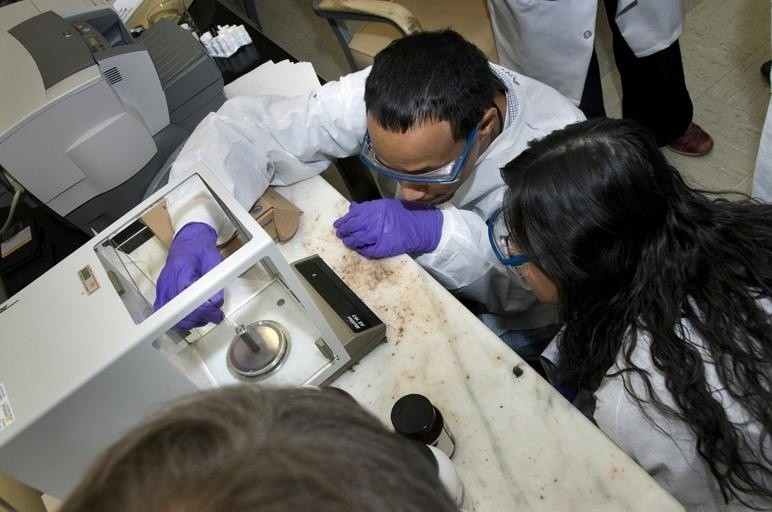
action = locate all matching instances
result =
[312,0,499,74]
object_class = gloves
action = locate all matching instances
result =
[333,198,443,260]
[154,222,223,330]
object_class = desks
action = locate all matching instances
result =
[269,173,689,511]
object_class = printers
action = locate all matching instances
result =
[0,0,231,237]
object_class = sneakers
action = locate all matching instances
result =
[666,122,713,157]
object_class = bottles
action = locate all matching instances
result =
[389,394,456,459]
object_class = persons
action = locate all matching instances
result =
[153,24,587,402]
[486,117,772,511]
[485,1,716,159]
[56,385,461,511]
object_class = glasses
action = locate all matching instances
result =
[486,207,545,267]
[359,119,480,186]
